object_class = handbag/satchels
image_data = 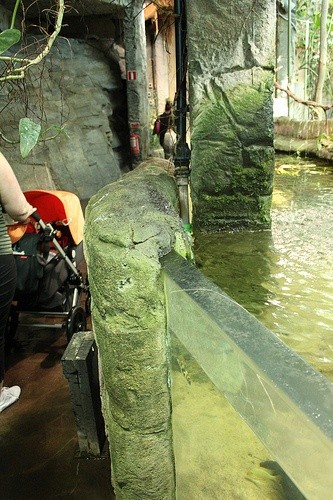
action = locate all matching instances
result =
[154,116,161,135]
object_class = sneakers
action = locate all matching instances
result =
[0,385,22,413]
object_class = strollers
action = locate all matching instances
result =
[5,189,91,345]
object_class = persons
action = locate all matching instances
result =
[0,150,37,410]
[152,102,172,148]
